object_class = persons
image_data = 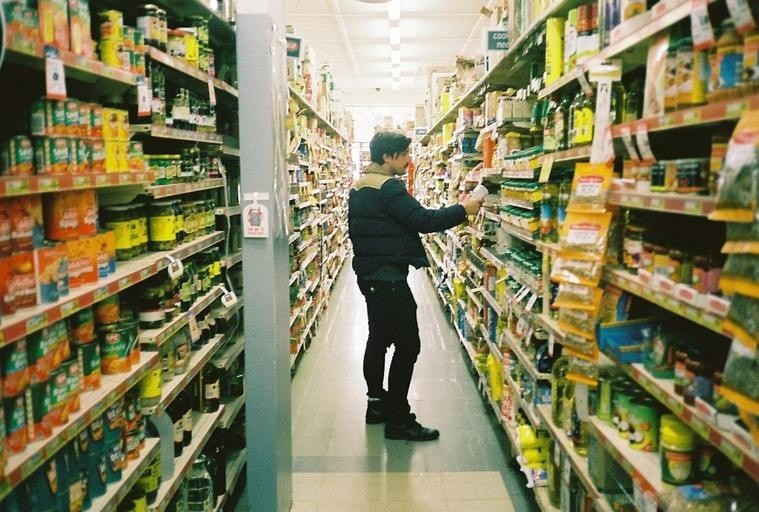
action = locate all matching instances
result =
[345,129,487,444]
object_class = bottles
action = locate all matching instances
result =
[487,346,514,421]
[538,178,575,245]
[656,413,727,486]
[153,81,223,182]
[542,81,644,154]
[498,145,544,230]
[136,246,227,512]
[711,17,743,99]
[483,262,496,298]
[550,349,589,456]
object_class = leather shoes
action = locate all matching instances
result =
[365,406,387,424]
[387,420,440,440]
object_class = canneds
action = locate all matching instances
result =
[675,38,707,109]
[622,158,717,196]
[622,225,721,295]
[0,4,222,512]
[598,372,665,499]
[664,44,675,113]
